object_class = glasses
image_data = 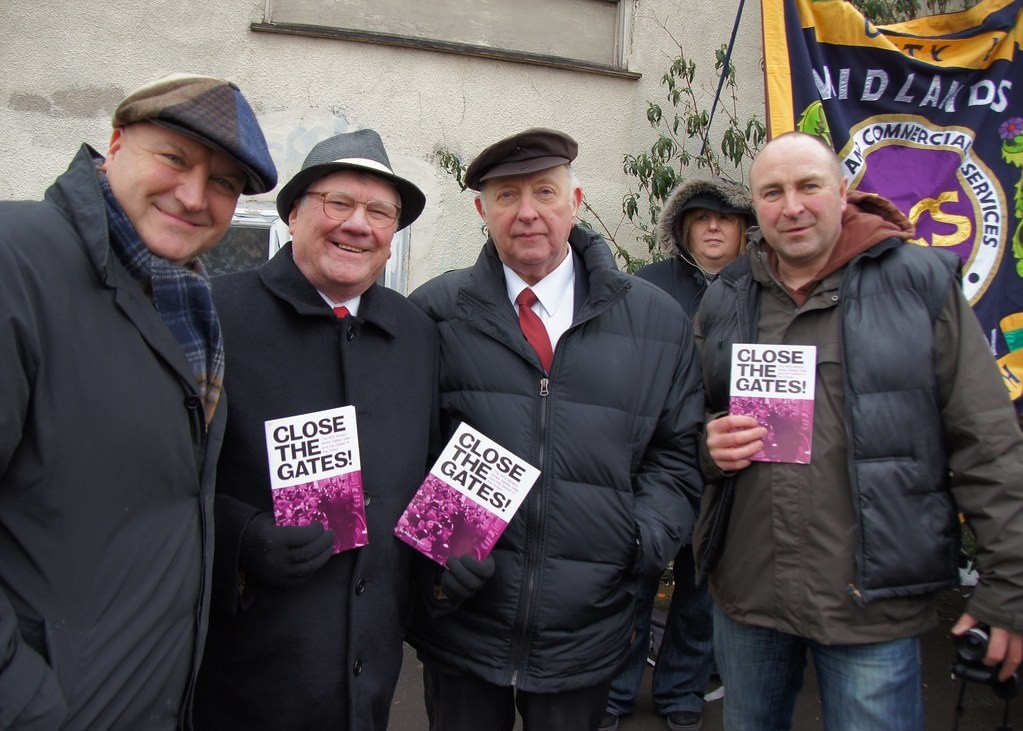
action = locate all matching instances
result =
[302,190,402,229]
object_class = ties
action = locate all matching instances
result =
[516,287,553,376]
[330,306,347,318]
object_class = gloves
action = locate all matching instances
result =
[416,552,496,612]
[235,515,335,591]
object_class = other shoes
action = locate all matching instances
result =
[668,712,701,731]
[599,716,618,730]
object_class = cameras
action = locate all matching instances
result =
[943,625,1022,691]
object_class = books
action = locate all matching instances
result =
[727,343,817,464]
[393,421,542,573]
[264,405,369,556]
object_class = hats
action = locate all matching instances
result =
[111,78,280,194]
[462,128,578,191]
[275,128,428,227]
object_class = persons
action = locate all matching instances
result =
[188,128,495,731]
[0,79,278,731]
[272,479,354,553]
[404,127,705,731]
[730,396,812,463]
[690,130,1023,731]
[595,176,759,731]
[395,475,486,567]
